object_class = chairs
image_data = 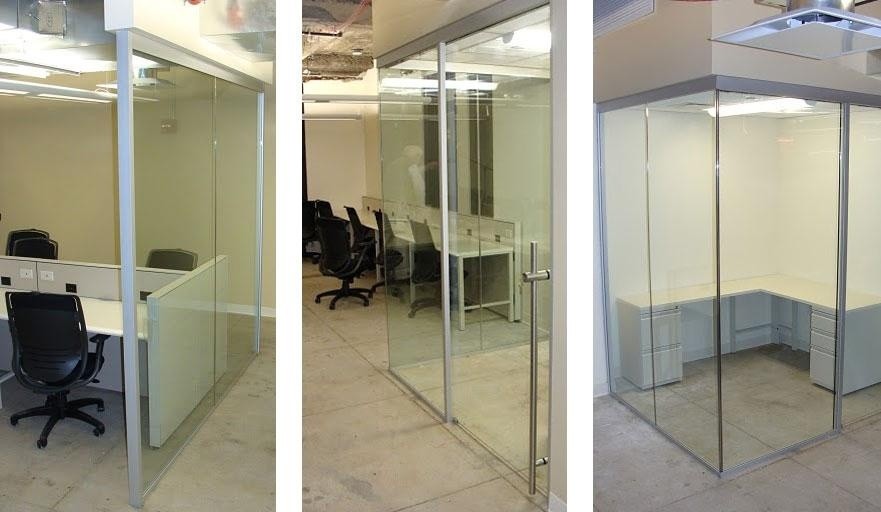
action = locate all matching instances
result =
[146,249,198,271]
[6,228,59,260]
[303,200,378,310]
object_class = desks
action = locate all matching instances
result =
[334,196,521,334]
[619,273,881,398]
